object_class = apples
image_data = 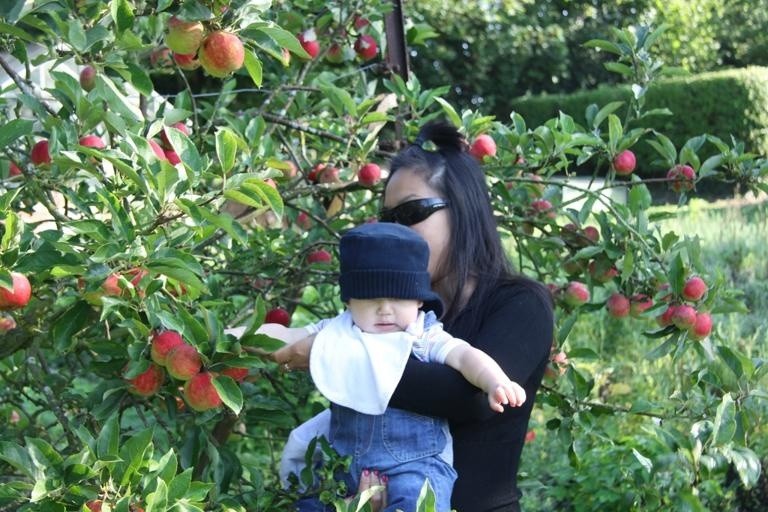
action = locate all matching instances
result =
[80,499,144,512]
[1,12,713,411]
[212,416,246,440]
[526,431,536,440]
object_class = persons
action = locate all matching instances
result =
[223,121,552,511]
[271,223,527,512]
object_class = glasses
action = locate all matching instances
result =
[377,198,449,227]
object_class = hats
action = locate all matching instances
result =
[340,223,443,320]
